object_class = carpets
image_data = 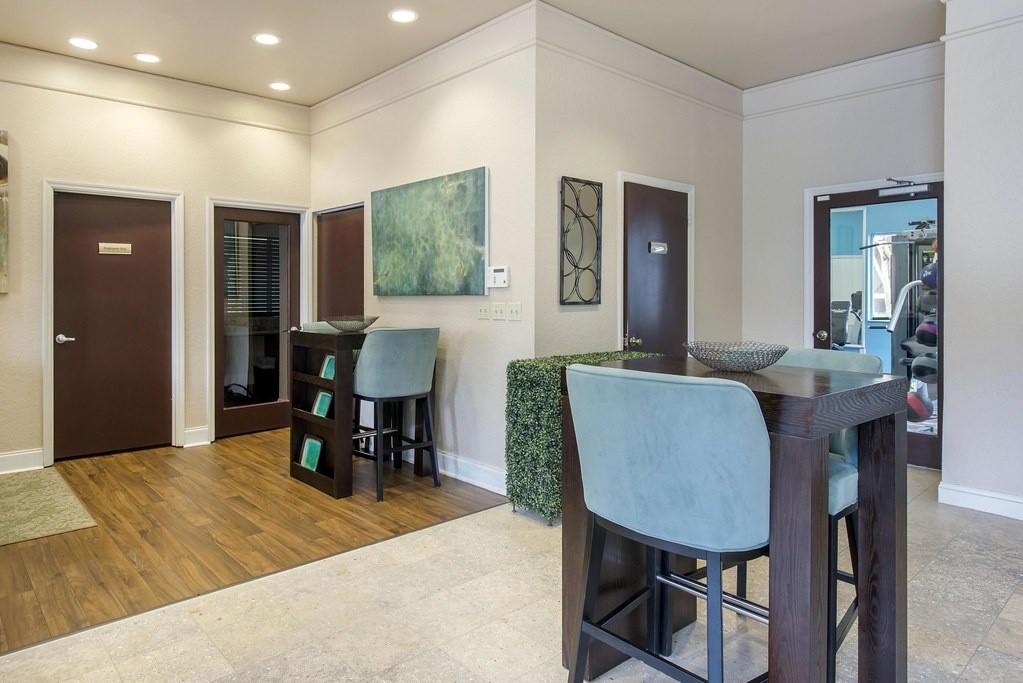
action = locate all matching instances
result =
[0,467,98,546]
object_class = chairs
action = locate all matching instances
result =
[566,363,771,683]
[352,326,442,503]
[303,320,382,461]
[723,346,883,683]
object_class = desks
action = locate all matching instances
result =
[289,327,438,499]
[504,349,666,524]
[560,356,909,683]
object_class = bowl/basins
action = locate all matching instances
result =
[682,340,790,373]
[322,316,379,333]
